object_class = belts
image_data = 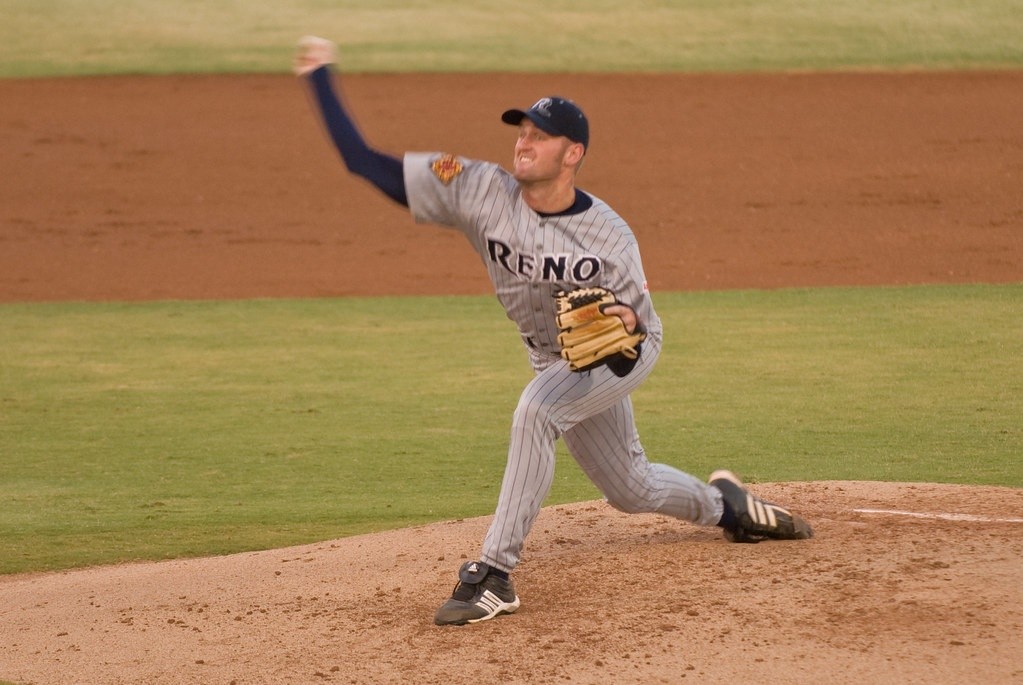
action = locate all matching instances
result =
[524,338,561,356]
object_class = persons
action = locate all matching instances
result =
[295,38,812,624]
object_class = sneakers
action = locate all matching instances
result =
[709,470,813,543]
[435,560,520,626]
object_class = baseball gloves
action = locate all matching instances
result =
[554,286,647,372]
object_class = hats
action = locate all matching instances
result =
[502,97,589,156]
